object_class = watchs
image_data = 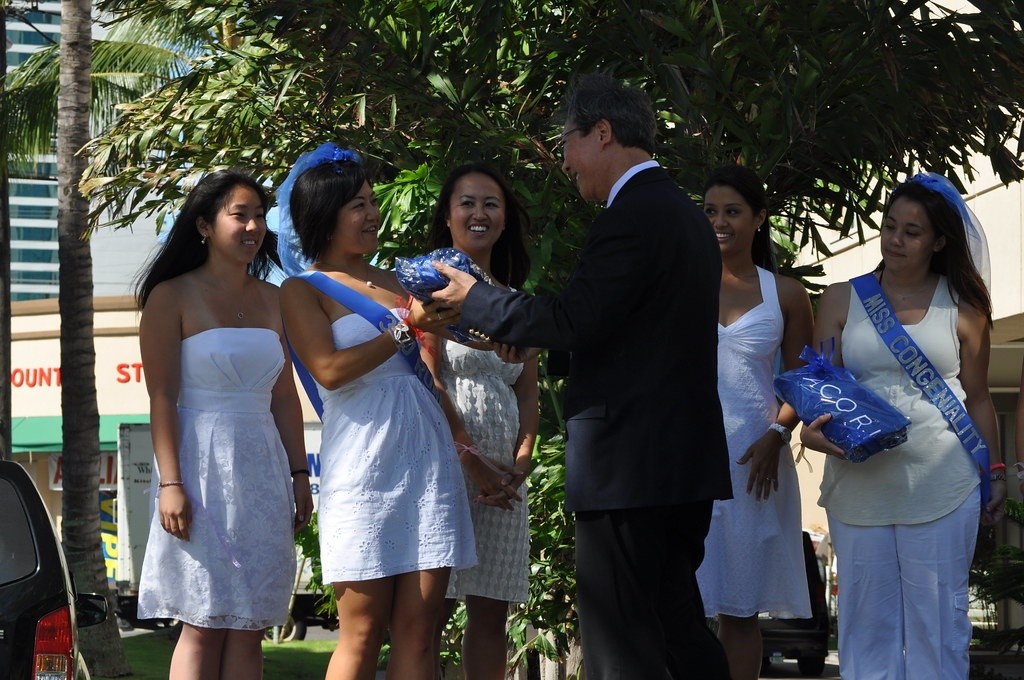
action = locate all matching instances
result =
[767,423,792,445]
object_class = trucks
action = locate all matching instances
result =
[118,421,339,644]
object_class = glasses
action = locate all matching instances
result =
[555,125,582,150]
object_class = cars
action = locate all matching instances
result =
[0,459,107,680]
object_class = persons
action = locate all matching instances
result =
[278,160,536,680]
[1014,357,1024,496]
[801,182,1007,680]
[694,168,815,680]
[135,170,314,680]
[426,76,734,679]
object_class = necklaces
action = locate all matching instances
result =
[237,312,244,319]
[319,264,373,287]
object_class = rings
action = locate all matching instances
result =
[438,313,444,321]
[766,478,770,481]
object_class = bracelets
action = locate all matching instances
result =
[1013,462,1024,490]
[291,470,309,477]
[981,463,1007,481]
[393,323,415,348]
[159,481,184,487]
